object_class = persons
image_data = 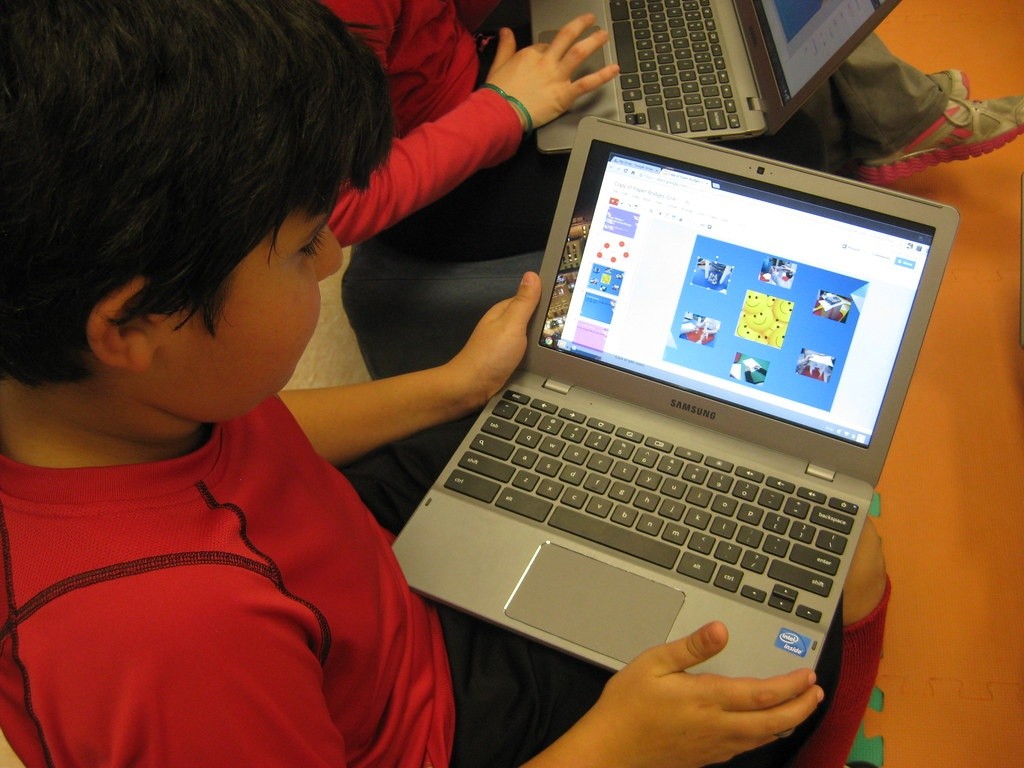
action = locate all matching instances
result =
[0,0,891,768]
[326,0,1024,247]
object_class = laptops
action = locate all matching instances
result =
[532,0,902,155]
[393,116,960,679]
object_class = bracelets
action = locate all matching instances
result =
[479,83,532,141]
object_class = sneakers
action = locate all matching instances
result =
[926,70,970,101]
[854,95,1024,186]
